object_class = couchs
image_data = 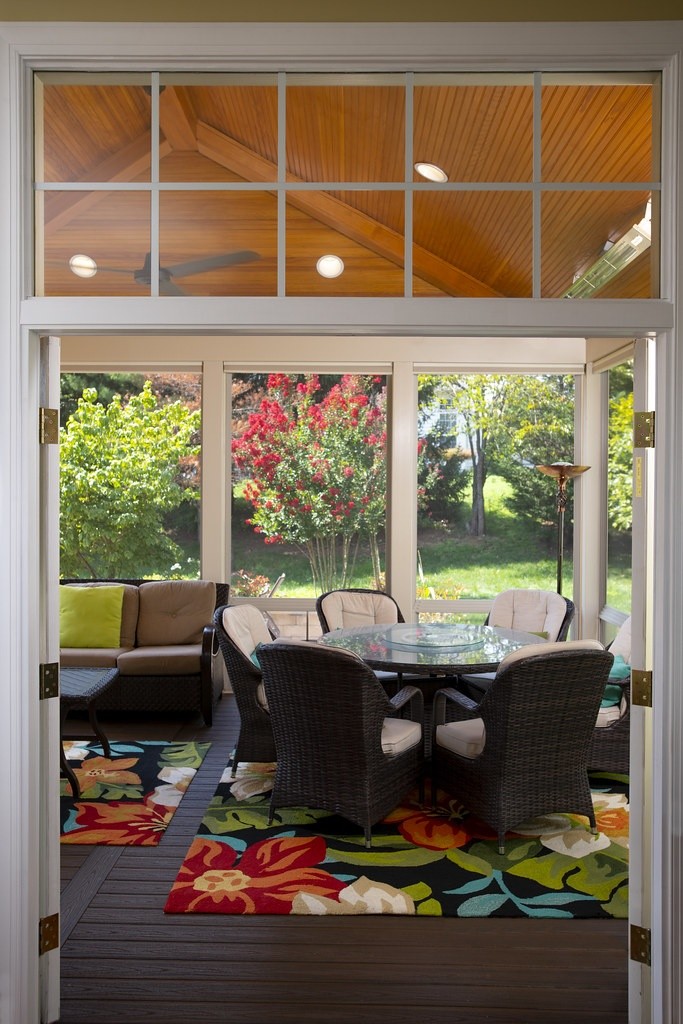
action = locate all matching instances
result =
[58,577,240,718]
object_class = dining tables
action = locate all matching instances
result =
[314,619,552,720]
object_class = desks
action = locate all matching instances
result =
[60,666,123,806]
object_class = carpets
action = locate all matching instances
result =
[162,734,630,922]
[59,736,211,850]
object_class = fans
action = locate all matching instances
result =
[44,250,263,295]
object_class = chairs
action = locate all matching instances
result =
[212,599,281,775]
[315,588,418,718]
[583,614,633,778]
[429,638,616,854]
[455,585,576,699]
[256,637,426,850]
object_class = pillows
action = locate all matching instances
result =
[59,584,126,650]
